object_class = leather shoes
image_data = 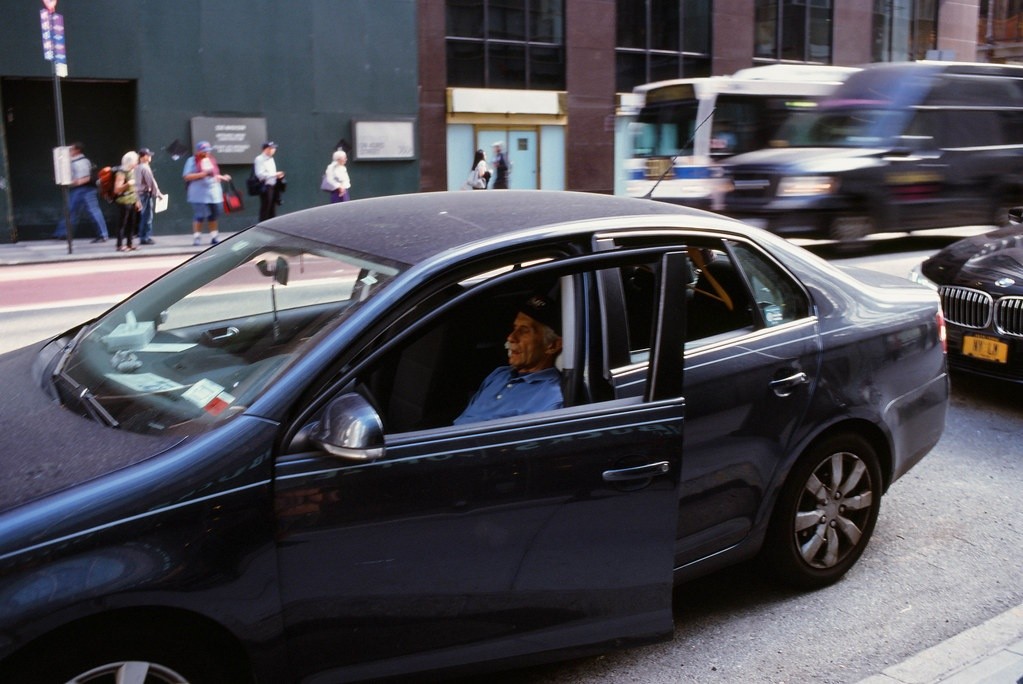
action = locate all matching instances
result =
[91,237,109,243]
[141,238,155,244]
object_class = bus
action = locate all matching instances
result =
[623,61,869,215]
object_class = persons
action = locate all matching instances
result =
[112,150,144,252]
[326,150,352,204]
[253,140,284,221]
[439,291,568,427]
[471,149,493,190]
[133,147,164,245]
[48,140,111,245]
[492,143,510,189]
[180,140,232,246]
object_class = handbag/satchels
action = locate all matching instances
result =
[320,166,341,191]
[246,158,267,195]
[467,162,486,188]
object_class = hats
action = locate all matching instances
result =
[197,141,215,153]
[137,148,155,156]
[506,289,562,335]
[263,141,279,150]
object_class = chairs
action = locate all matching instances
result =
[359,283,476,434]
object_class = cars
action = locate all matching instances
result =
[909,219,1023,404]
[0,187,952,684]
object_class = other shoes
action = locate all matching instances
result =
[192,236,201,247]
[128,244,137,251]
[116,245,129,251]
[210,237,220,243]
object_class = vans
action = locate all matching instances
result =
[724,58,1023,249]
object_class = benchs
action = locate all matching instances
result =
[622,258,754,352]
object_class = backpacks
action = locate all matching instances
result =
[94,165,131,204]
